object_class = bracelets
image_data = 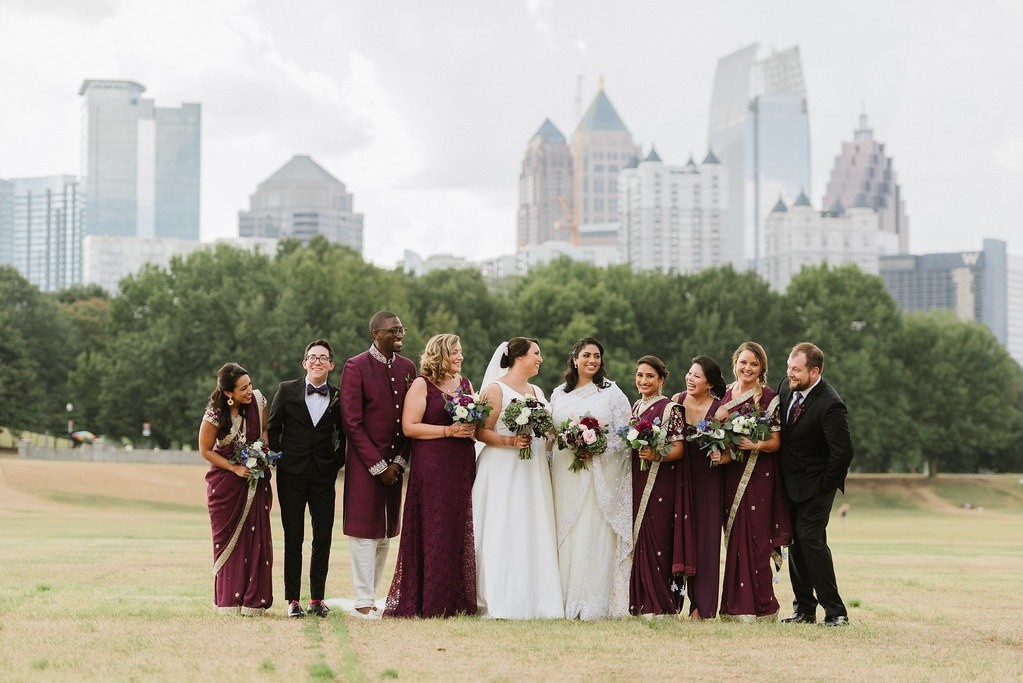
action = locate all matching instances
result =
[444,425,453,437]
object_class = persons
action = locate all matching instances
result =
[339,310,417,620]
[776,342,854,626]
[546,337,632,620]
[383,334,475,617]
[472,336,565,619]
[268,339,345,619]
[199,362,273,617]
[670,355,739,621]
[631,355,687,619]
[718,342,794,621]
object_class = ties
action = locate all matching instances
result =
[787,391,803,427]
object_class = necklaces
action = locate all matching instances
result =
[230,417,246,444]
[506,373,528,392]
[440,376,457,393]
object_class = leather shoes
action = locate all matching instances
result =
[288,601,306,618]
[818,615,850,627]
[307,601,327,618]
[781,612,815,623]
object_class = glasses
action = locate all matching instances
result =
[375,327,407,335]
[301,355,331,367]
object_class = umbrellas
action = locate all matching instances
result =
[71,430,97,443]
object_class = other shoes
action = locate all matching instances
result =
[376,608,385,615]
[351,608,381,621]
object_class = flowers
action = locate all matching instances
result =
[405,372,413,390]
[794,403,805,421]
[685,415,744,468]
[727,403,774,463]
[228,432,282,490]
[555,415,609,474]
[329,389,339,412]
[501,396,553,461]
[442,384,495,434]
[614,416,673,471]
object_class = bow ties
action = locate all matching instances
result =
[307,383,328,397]
